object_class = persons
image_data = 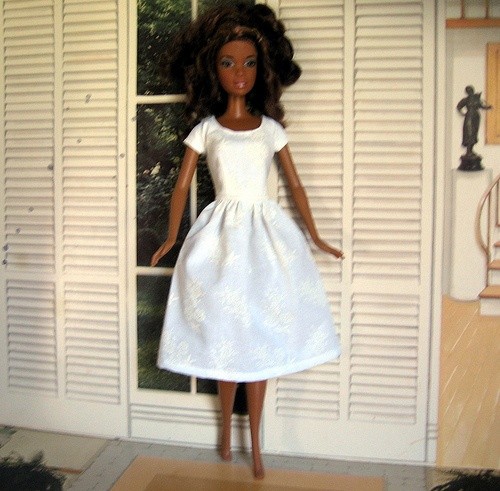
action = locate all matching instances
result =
[457,86,493,154]
[150,1,344,480]
